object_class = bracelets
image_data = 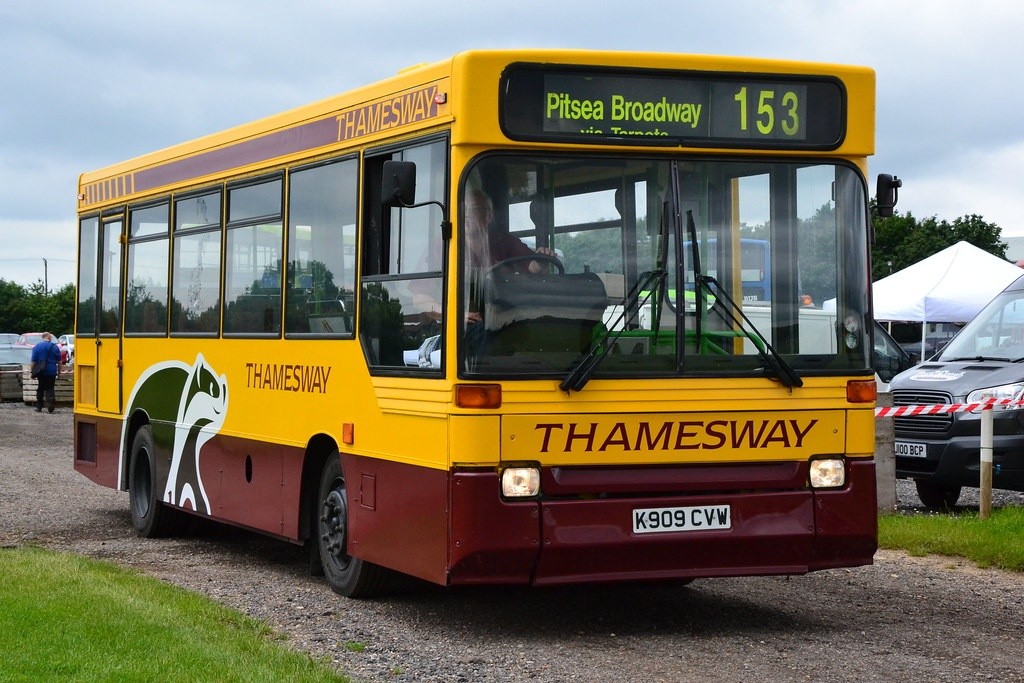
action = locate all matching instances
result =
[30,372,32,373]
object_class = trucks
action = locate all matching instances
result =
[603,303,919,395]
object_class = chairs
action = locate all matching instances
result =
[186,287,374,365]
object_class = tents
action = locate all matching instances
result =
[822,239,1024,366]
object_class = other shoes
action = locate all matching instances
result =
[48,407,54,413]
[34,406,41,411]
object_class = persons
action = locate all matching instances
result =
[29,332,63,413]
[405,187,560,327]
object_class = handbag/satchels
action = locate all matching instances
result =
[30,343,55,378]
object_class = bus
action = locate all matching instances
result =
[72,51,903,598]
[674,233,773,301]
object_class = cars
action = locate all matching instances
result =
[0,332,75,363]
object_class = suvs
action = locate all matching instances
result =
[888,272,1024,512]
